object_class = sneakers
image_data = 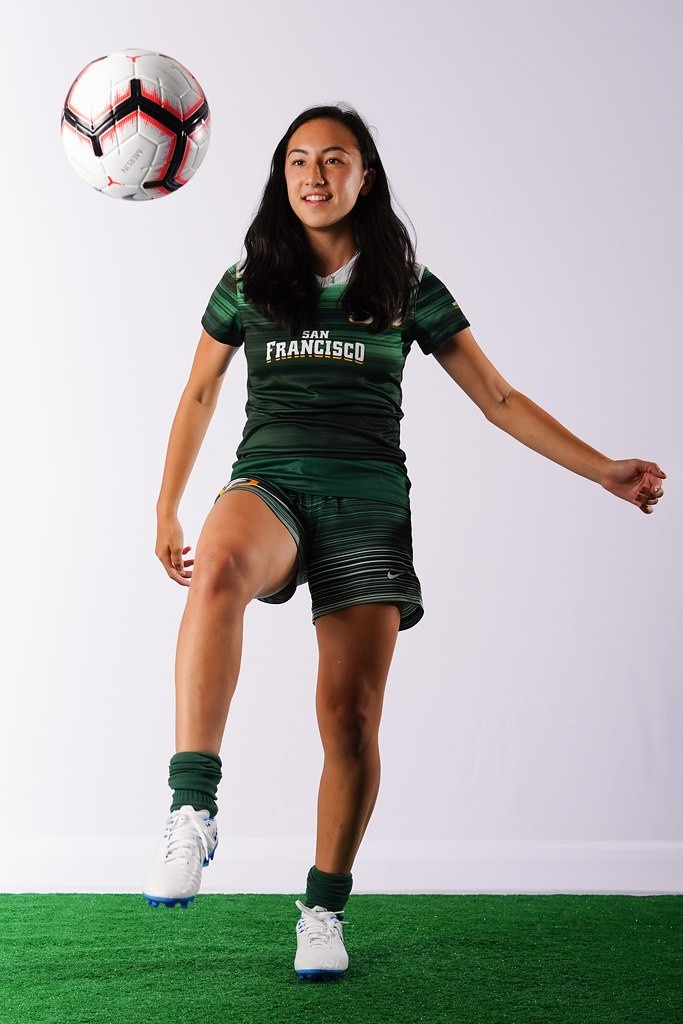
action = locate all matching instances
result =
[291,906,350,982]
[144,805,218,909]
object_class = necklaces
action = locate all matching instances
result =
[313,248,360,284]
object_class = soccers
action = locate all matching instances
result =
[60,49,210,202]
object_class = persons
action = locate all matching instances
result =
[139,104,667,981]
